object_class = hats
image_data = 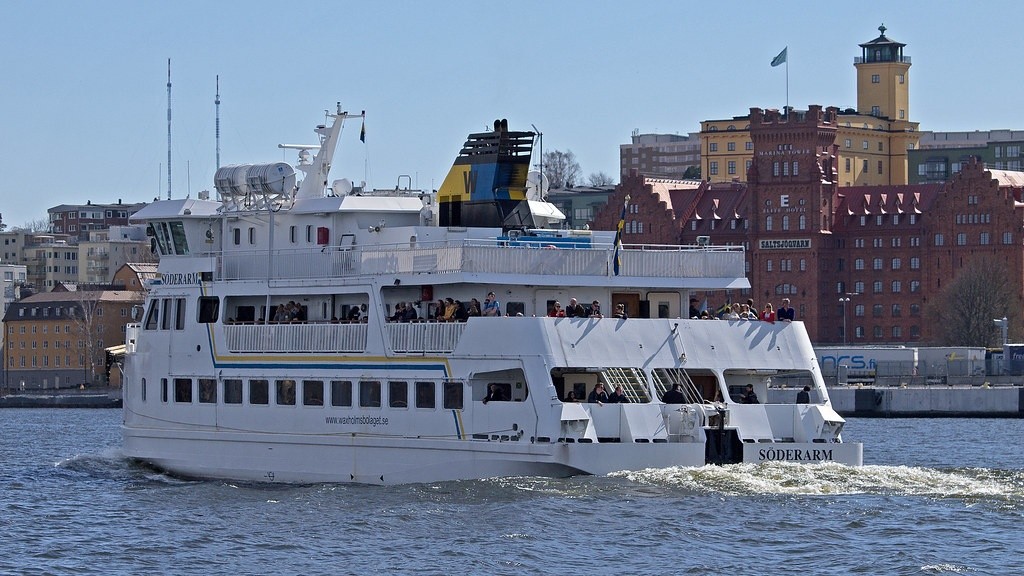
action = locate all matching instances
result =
[673,382,683,386]
[690,298,699,303]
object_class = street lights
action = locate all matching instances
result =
[839,298,850,347]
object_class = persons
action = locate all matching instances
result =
[503,311,535,317]
[273,301,306,324]
[550,298,603,318]
[689,298,760,321]
[777,298,795,323]
[548,245,560,250]
[760,303,774,323]
[662,383,687,404]
[481,292,501,317]
[482,383,502,404]
[331,306,368,325]
[739,383,760,404]
[714,391,724,402]
[227,318,264,325]
[796,386,811,404]
[385,298,482,323]
[614,304,628,320]
[562,383,629,407]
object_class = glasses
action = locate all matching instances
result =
[557,306,560,307]
[595,305,600,307]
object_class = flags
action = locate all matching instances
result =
[699,296,707,315]
[771,46,786,67]
[716,297,731,318]
[613,202,624,277]
[360,122,364,143]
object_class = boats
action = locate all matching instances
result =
[119,100,864,487]
